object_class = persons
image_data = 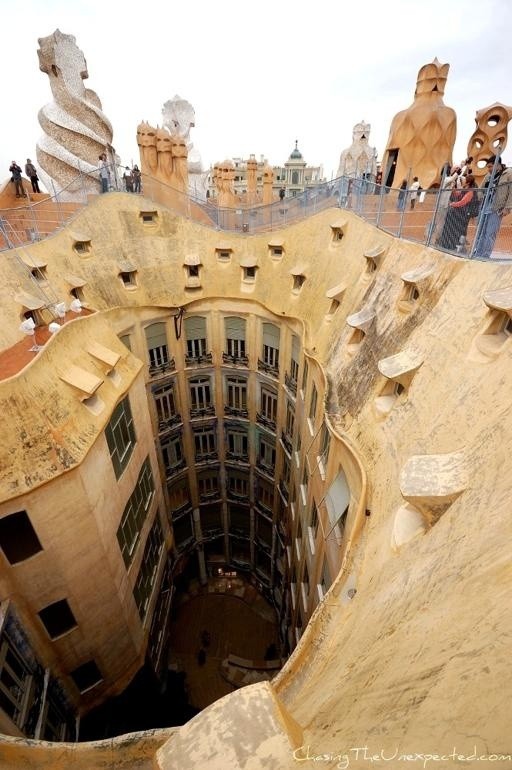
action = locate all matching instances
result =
[8,160,27,199]
[470,154,511,265]
[97,153,109,193]
[131,164,142,194]
[408,176,420,210]
[122,166,135,193]
[396,179,407,210]
[435,174,475,252]
[24,158,42,193]
[102,154,111,175]
